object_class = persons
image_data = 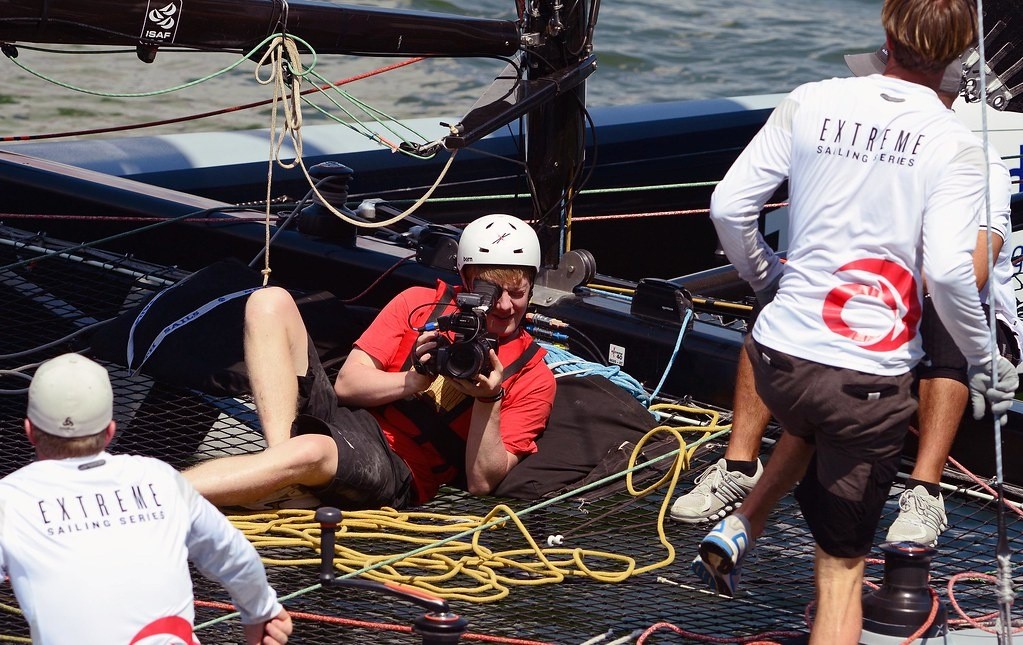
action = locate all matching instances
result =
[669,0,1022,645]
[0,352,292,645]
[180,214,556,510]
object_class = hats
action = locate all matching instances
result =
[26,353,114,438]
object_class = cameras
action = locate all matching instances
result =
[423,279,502,383]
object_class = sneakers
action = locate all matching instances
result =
[885,484,948,549]
[690,515,748,600]
[670,456,764,523]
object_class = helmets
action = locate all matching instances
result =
[457,214,541,273]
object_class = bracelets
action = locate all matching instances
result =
[476,387,505,403]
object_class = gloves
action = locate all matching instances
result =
[754,272,784,309]
[966,356,1019,426]
